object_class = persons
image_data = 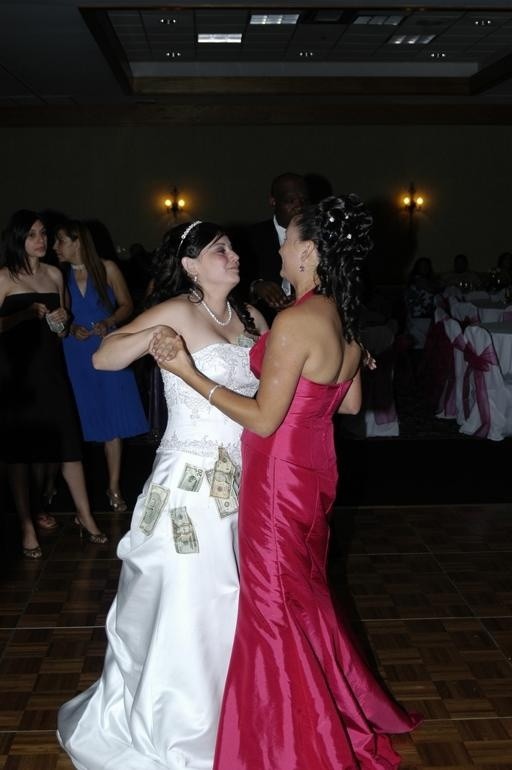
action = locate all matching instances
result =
[403,252,512,319]
[229,171,315,331]
[34,218,151,533]
[2,210,113,563]
[145,193,426,770]
[49,219,276,770]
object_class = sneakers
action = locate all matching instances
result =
[38,513,56,528]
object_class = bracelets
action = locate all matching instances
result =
[208,384,226,406]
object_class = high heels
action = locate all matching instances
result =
[108,488,127,511]
[23,545,41,558]
[75,514,108,543]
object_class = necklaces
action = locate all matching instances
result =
[190,289,233,327]
